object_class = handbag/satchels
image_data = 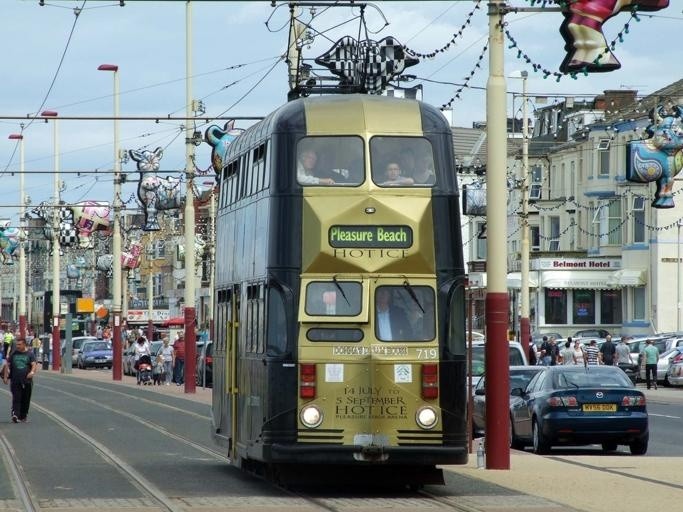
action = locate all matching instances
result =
[580,347,588,364]
[0,352,11,379]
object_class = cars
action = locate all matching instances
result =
[22,327,213,388]
[462,325,683,456]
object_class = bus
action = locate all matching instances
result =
[204,93,471,486]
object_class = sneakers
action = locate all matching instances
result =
[135,380,182,387]
[12,414,29,423]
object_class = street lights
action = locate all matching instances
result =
[6,133,28,336]
[510,68,531,363]
[40,109,63,370]
[98,62,123,382]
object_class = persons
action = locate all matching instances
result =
[155,337,174,386]
[132,336,150,386]
[411,155,435,186]
[0,319,41,378]
[152,354,163,386]
[349,286,414,342]
[94,324,144,351]
[508,330,660,392]
[373,161,414,189]
[295,150,335,185]
[173,329,184,387]
[1,337,38,424]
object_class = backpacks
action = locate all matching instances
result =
[529,343,537,365]
